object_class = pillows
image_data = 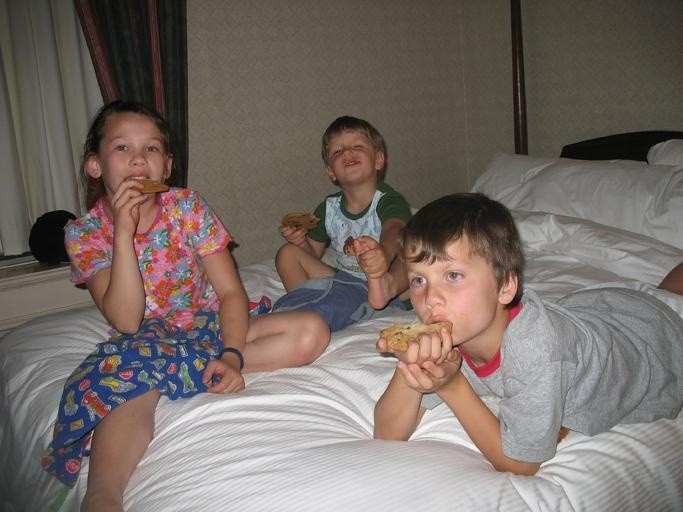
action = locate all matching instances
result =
[468,139,683,250]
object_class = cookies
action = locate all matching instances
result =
[378,318,454,355]
[281,211,321,230]
[125,177,170,195]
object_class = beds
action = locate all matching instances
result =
[0,130,683,512]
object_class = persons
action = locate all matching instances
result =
[275,115,413,312]
[64,103,331,512]
[375,191,683,474]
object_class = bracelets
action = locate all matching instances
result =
[219,346,244,368]
